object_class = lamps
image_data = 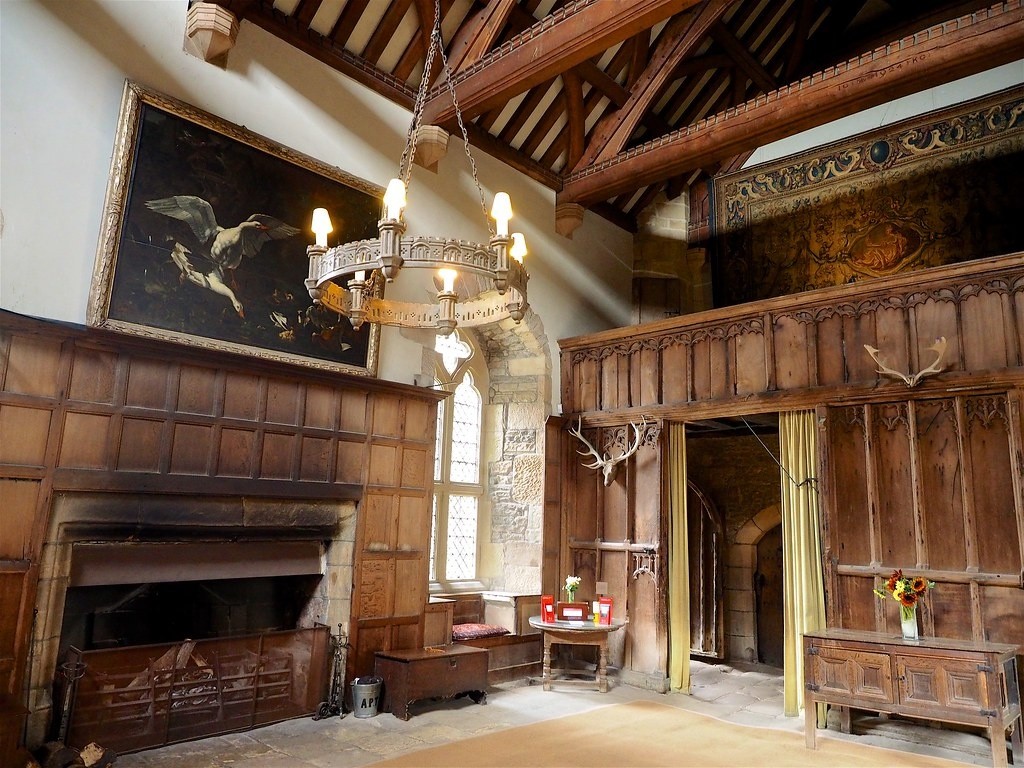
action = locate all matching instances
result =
[302,0,531,336]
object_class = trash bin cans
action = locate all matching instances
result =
[350,677,384,719]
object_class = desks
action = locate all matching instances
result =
[527,614,627,692]
[370,644,492,722]
[800,628,1024,768]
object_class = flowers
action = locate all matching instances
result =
[561,575,582,602]
[871,567,936,621]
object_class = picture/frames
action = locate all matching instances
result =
[84,78,387,377]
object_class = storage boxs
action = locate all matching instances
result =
[599,597,613,624]
[541,595,554,622]
[544,607,555,622]
[557,601,588,620]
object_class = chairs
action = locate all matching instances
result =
[435,592,520,647]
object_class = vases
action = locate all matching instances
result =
[899,604,919,641]
